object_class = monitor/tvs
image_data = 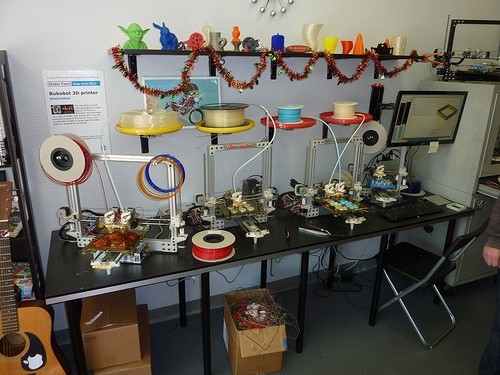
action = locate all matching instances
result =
[385,90,468,147]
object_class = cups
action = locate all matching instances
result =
[392,35,407,55]
[322,37,338,54]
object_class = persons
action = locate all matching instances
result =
[475,194,500,375]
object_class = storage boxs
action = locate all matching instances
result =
[80,289,141,370]
[94,304,151,375]
[223,289,288,375]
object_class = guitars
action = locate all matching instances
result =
[0,181,72,375]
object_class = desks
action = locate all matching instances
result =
[44,189,472,375]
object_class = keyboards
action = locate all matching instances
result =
[377,200,444,222]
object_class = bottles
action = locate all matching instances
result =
[207,32,227,51]
[271,33,284,53]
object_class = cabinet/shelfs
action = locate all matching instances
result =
[0,51,56,346]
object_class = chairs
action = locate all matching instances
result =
[374,216,491,350]
